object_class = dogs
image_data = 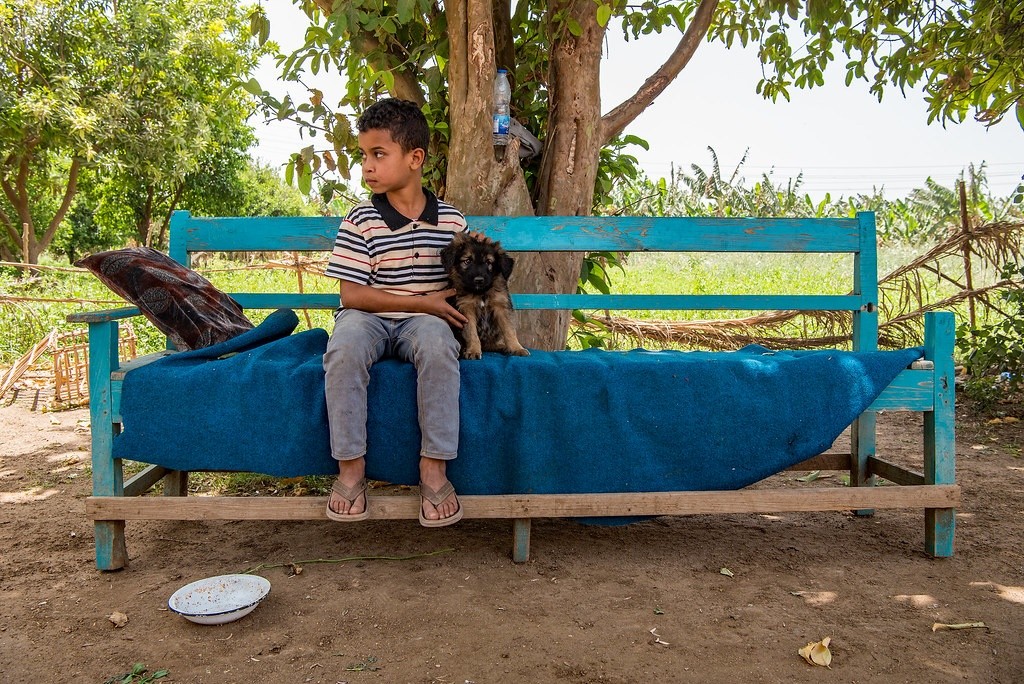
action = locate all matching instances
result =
[439,231,531,360]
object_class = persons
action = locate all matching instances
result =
[323,96,493,528]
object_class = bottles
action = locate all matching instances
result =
[493,70,511,145]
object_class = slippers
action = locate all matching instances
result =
[419,480,463,526]
[326,477,369,521]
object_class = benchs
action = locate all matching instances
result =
[66,210,960,571]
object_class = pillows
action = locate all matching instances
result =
[73,246,257,353]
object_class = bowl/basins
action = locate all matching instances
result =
[168,574,270,624]
